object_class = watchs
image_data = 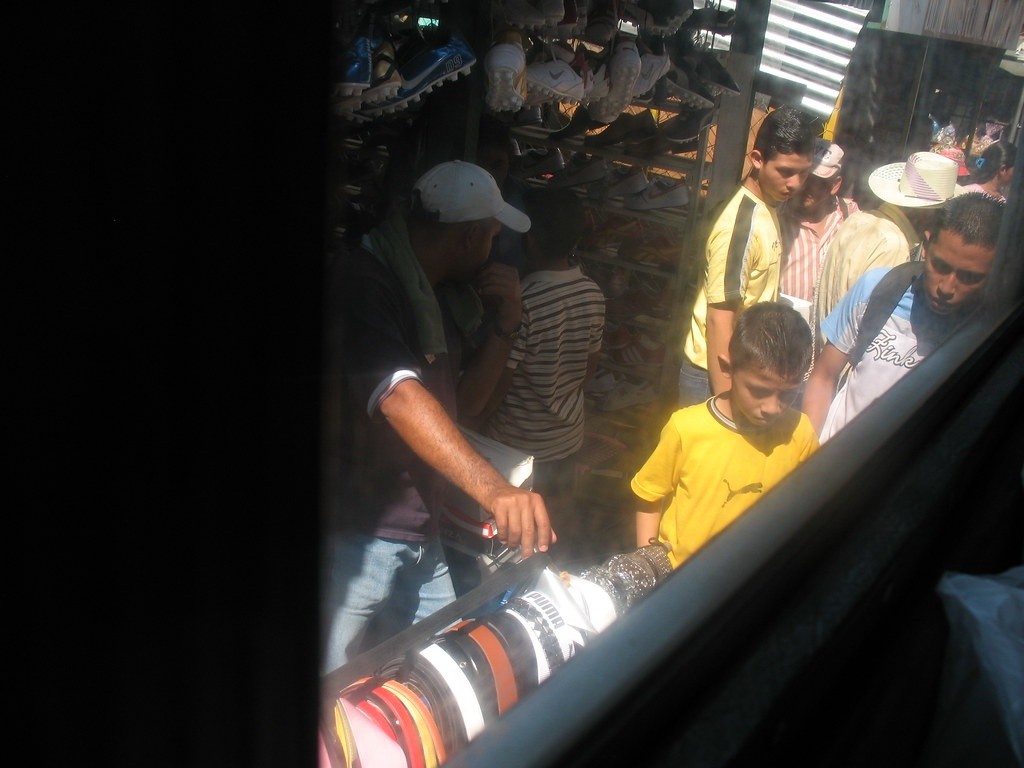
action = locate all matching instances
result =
[492,321,524,341]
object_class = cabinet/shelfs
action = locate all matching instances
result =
[324,3,718,515]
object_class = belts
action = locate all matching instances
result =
[319,591,577,768]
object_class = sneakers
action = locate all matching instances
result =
[484,0,737,476]
[328,22,477,125]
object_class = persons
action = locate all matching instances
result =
[675,104,1019,445]
[631,302,821,572]
[482,190,605,553]
[322,161,558,671]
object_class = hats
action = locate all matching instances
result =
[411,159,531,233]
[868,152,965,209]
[930,144,970,176]
[809,143,844,178]
